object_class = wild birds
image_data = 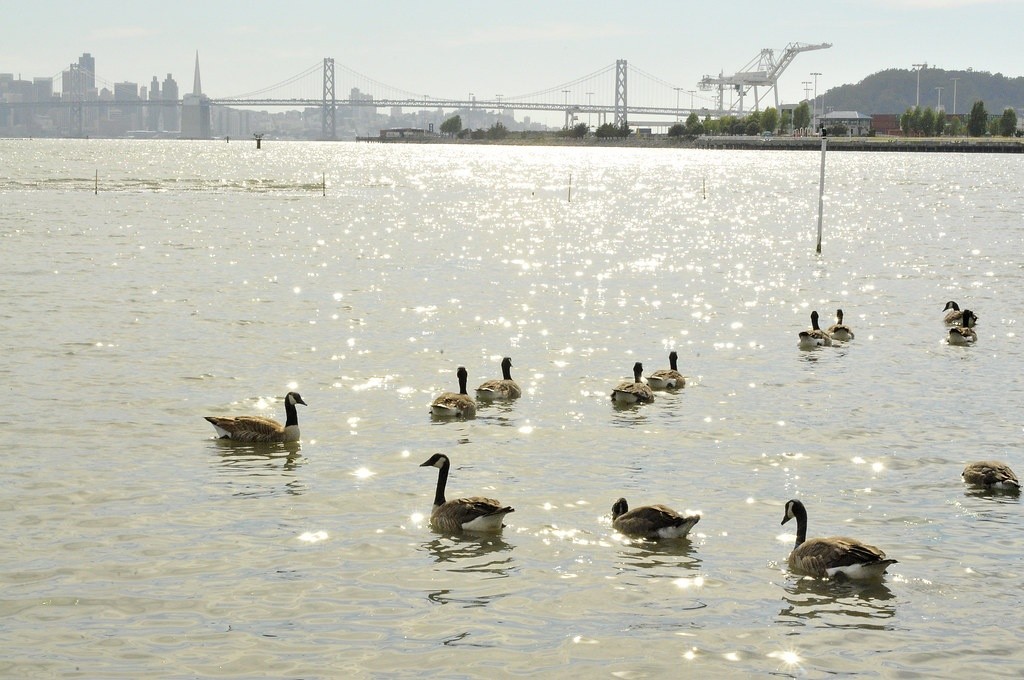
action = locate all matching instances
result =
[961,460,1023,489]
[429,366,477,417]
[644,351,686,389]
[781,499,901,582]
[611,498,700,539]
[203,392,307,442]
[942,301,979,326]
[609,361,654,403]
[827,309,854,340]
[474,357,521,399]
[420,453,515,532]
[946,309,977,346]
[797,311,832,347]
